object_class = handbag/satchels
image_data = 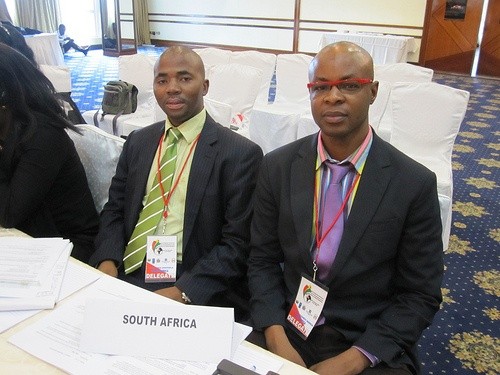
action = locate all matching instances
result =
[102,80,138,115]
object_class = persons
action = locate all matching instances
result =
[85,45,264,320]
[236,41,444,375]
[57,24,89,56]
[0,23,99,263]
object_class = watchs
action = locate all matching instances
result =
[181,291,192,304]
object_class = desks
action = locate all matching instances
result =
[317,33,416,64]
[0,229,315,375]
[21,34,67,69]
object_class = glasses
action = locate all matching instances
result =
[307,78,372,92]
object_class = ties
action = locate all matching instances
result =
[122,128,183,274]
[313,161,354,281]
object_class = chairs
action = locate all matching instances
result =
[64,46,471,252]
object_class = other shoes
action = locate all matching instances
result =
[84,47,90,56]
